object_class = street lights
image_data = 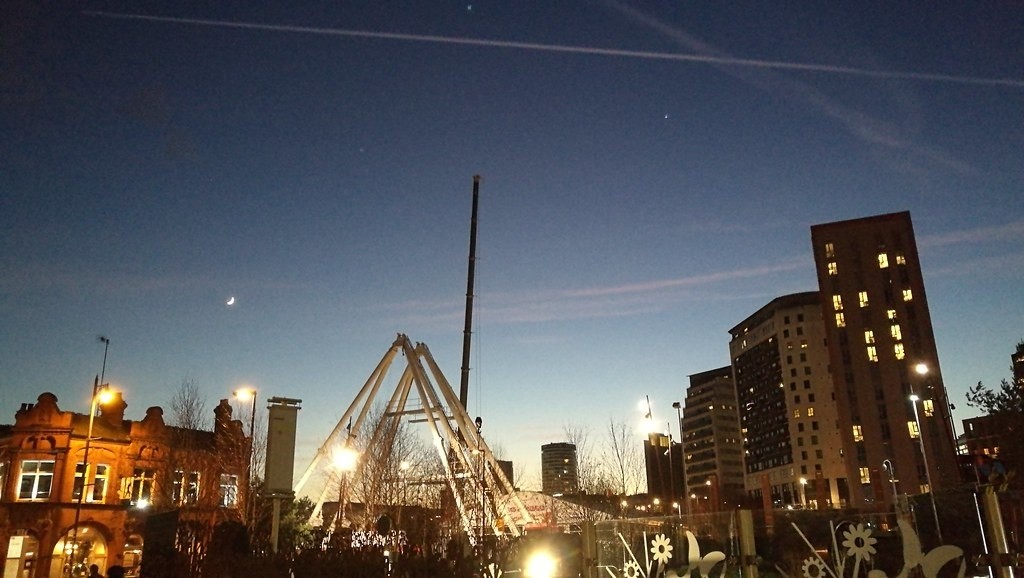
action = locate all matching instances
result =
[135,495,151,513]
[949,404,968,483]
[655,497,663,510]
[68,387,120,577]
[672,501,681,515]
[618,498,632,575]
[549,492,591,578]
[908,357,945,545]
[474,419,489,578]
[670,400,687,443]
[705,473,723,511]
[882,460,898,507]
[373,515,395,537]
[639,391,656,431]
[690,493,699,515]
[233,381,257,522]
[332,445,359,527]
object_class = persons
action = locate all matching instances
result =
[87,564,104,578]
